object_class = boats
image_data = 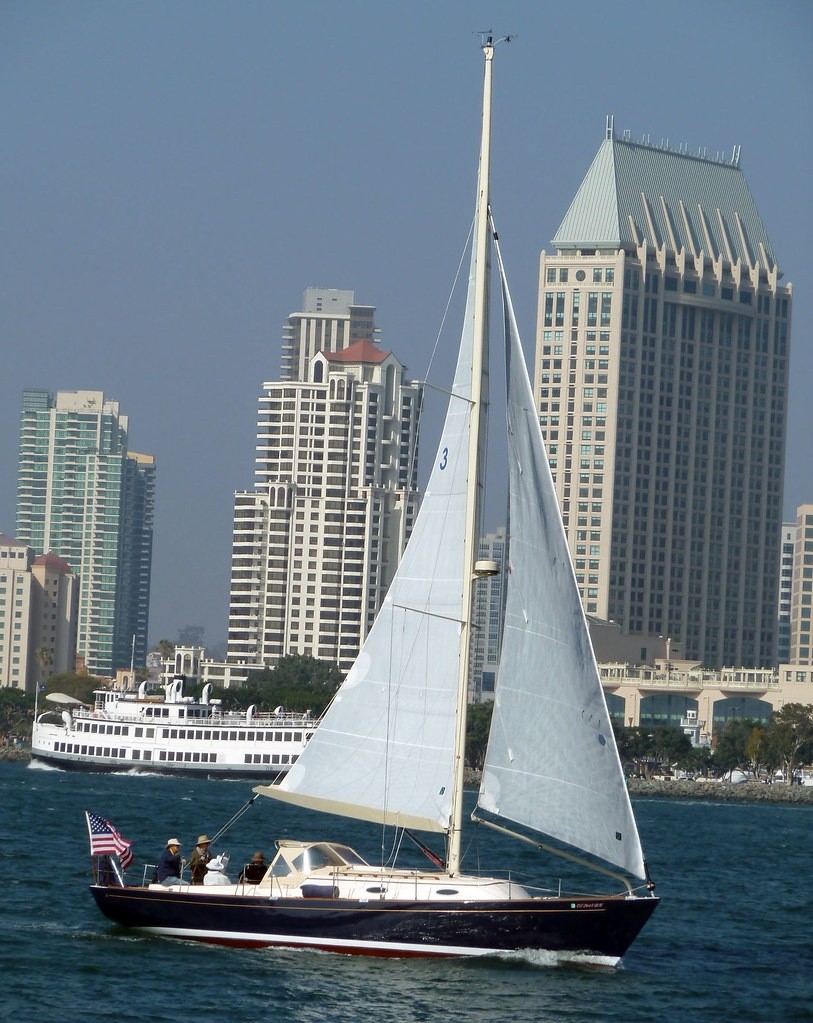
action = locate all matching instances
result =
[30,634,334,784]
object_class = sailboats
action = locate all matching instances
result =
[85,56,664,983]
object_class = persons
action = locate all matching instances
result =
[188,834,210,885]
[238,852,268,886]
[158,838,190,886]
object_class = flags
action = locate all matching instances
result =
[84,809,132,869]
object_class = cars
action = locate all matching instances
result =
[679,778,695,782]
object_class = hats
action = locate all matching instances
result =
[164,837,181,847]
[251,850,266,860]
[194,834,212,845]
[205,858,224,868]
